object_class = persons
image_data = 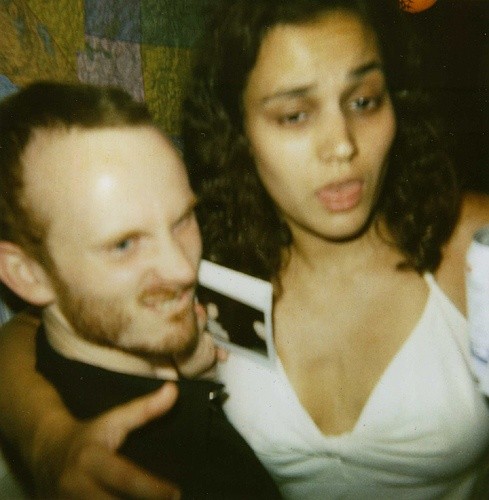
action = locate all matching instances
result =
[1,78,283,500]
[1,0,488,500]
